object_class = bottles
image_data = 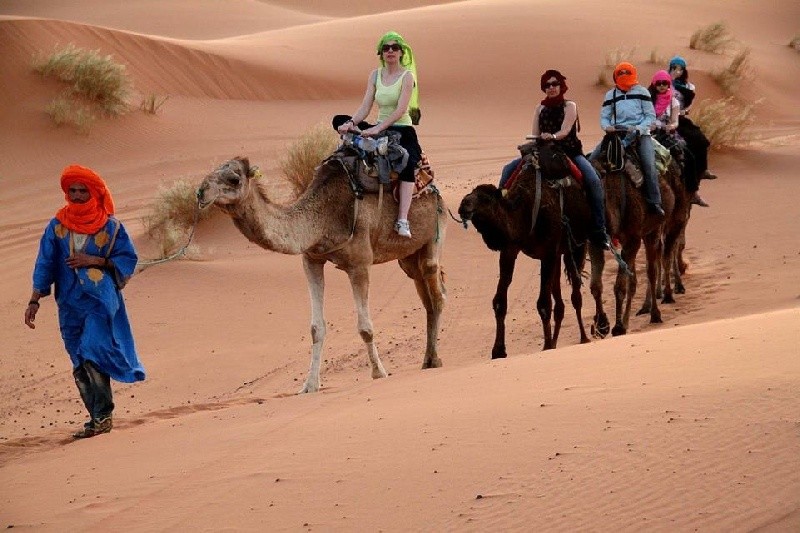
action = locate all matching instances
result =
[340,133,377,151]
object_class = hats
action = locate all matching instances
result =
[671,58,686,67]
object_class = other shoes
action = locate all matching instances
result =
[700,172,717,179]
[648,203,665,215]
[689,196,709,207]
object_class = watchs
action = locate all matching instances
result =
[553,134,556,140]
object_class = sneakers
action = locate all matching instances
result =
[592,227,611,250]
[393,221,412,238]
[376,137,388,154]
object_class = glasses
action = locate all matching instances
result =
[618,70,631,75]
[656,81,669,86]
[544,81,561,88]
[673,66,682,71]
[69,187,89,195]
[381,44,403,52]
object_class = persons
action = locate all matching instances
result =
[649,56,717,207]
[24,164,145,437]
[332,32,420,238]
[499,71,610,251]
[590,63,665,218]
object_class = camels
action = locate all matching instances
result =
[459,161,615,359]
[197,155,447,395]
[564,114,692,344]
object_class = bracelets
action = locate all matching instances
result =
[29,301,39,308]
[346,120,354,126]
[103,257,109,268]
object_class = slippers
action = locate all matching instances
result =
[93,414,113,434]
[72,420,94,438]
